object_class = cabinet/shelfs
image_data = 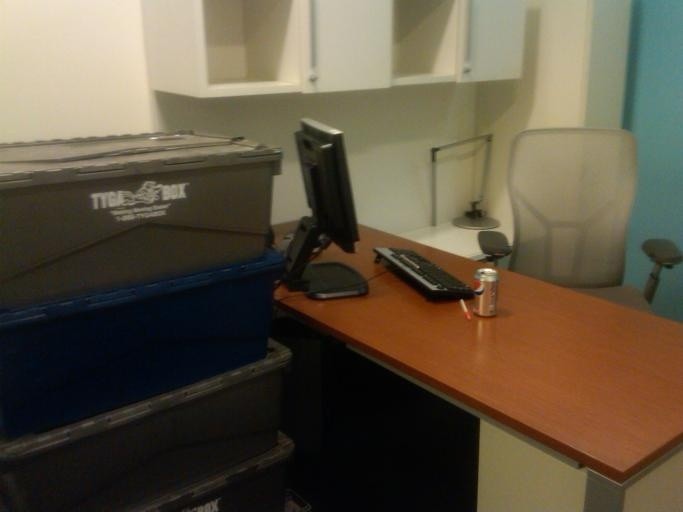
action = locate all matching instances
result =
[143,2,526,109]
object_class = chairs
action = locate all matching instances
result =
[478,128,683,316]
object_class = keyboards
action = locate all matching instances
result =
[372,247,473,302]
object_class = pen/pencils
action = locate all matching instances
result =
[460,299,471,320]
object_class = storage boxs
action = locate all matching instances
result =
[1,131,282,304]
[1,249,282,431]
[92,432,294,512]
[0,337,291,512]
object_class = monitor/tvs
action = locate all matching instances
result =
[280,117,369,300]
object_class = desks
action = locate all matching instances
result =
[271,217,683,511]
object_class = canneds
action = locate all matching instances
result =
[471,268,499,317]
